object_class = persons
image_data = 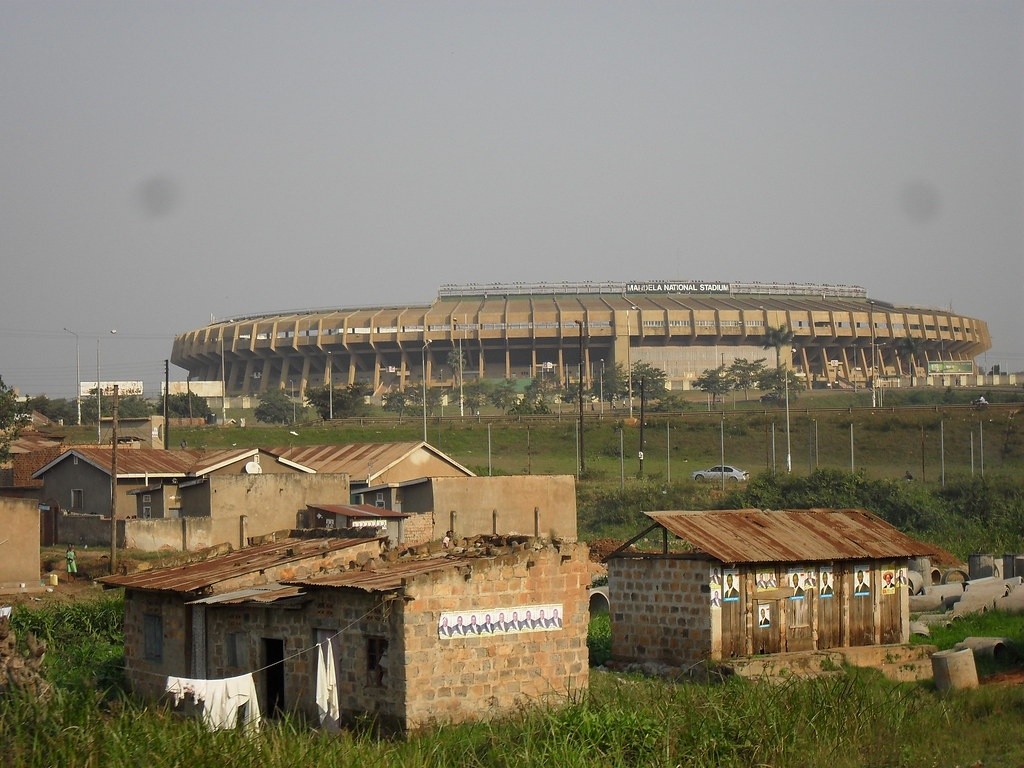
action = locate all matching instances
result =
[821,572,832,595]
[441,530,453,549]
[508,611,521,630]
[466,615,481,635]
[804,571,816,587]
[896,570,907,585]
[481,614,494,633]
[760,609,769,625]
[548,609,562,627]
[66,545,77,583]
[711,591,721,606]
[711,568,721,585]
[757,573,767,588]
[452,616,466,636]
[767,572,776,588]
[522,611,535,629]
[534,610,549,628]
[975,393,987,404]
[439,616,452,637]
[856,570,869,593]
[792,573,804,596]
[725,574,739,598]
[883,571,894,588]
[494,613,508,632]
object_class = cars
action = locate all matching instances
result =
[690,464,750,484]
[759,392,782,403]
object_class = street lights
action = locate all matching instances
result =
[96,329,118,446]
[453,317,465,418]
[63,327,81,424]
[626,305,637,419]
[869,300,879,410]
[327,351,333,420]
[574,320,587,472]
[421,338,433,442]
[869,342,887,407]
